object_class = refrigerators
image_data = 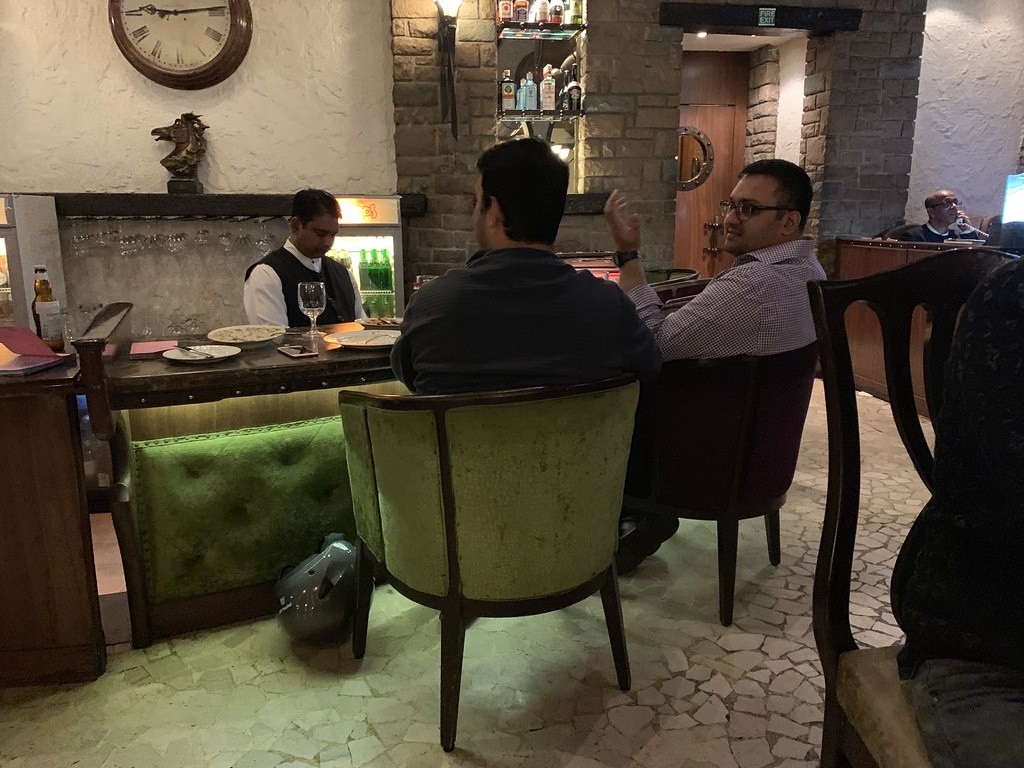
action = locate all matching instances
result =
[326,195,405,320]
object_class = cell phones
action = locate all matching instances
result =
[277,345,319,358]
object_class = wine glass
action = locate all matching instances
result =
[60,300,211,344]
[65,215,294,261]
[297,281,328,337]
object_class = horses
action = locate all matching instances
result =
[151,112,210,178]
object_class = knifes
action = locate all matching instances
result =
[173,344,214,359]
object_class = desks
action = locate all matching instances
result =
[73,303,425,652]
[0,349,108,687]
[832,234,1024,425]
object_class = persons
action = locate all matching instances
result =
[605,159,827,572]
[889,257,1024,768]
[390,138,667,391]
[906,190,978,242]
[243,188,368,328]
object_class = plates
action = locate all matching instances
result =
[323,330,401,349]
[162,345,240,364]
[207,324,286,348]
[356,317,405,329]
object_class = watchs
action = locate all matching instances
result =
[614,250,640,267]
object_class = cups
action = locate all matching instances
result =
[416,275,439,291]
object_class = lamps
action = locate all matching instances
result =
[434,0,464,143]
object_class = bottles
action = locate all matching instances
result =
[496,0,584,26]
[501,63,582,112]
[31,264,65,350]
[325,247,394,318]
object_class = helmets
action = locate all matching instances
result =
[274,532,376,646]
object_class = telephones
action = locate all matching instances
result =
[956,217,963,225]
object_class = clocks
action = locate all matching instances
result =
[108,0,253,91]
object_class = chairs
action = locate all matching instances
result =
[805,251,1024,768]
[637,336,821,626]
[643,269,714,317]
[339,369,642,753]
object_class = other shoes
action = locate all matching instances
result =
[614,514,680,577]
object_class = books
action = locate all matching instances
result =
[130,340,178,359]
[0,327,76,375]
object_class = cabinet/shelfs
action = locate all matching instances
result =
[496,0,587,122]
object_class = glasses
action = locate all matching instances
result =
[933,199,963,208]
[718,199,794,221]
